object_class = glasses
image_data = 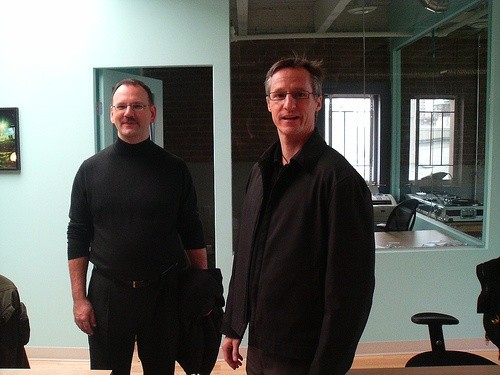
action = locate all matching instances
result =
[267,91,320,100]
[112,103,153,111]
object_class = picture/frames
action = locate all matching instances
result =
[0,108,21,173]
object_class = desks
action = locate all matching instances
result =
[346,366,500,375]
[374,230,465,250]
[0,369,113,375]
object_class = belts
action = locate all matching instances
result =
[94,262,178,289]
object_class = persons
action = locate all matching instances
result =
[67,77,212,375]
[220,54,375,375]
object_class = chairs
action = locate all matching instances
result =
[0,274,31,370]
[374,199,420,231]
